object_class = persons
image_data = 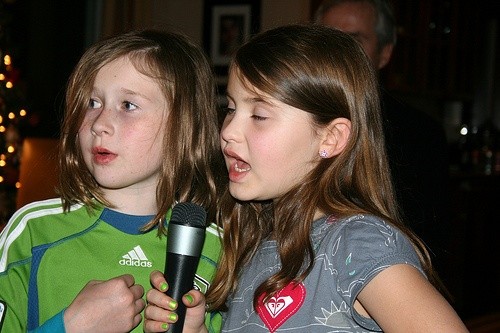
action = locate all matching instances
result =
[301,0,496,326]
[0,26,239,333]
[216,15,241,57]
[142,24,474,333]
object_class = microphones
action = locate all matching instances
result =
[159,203,206,333]
